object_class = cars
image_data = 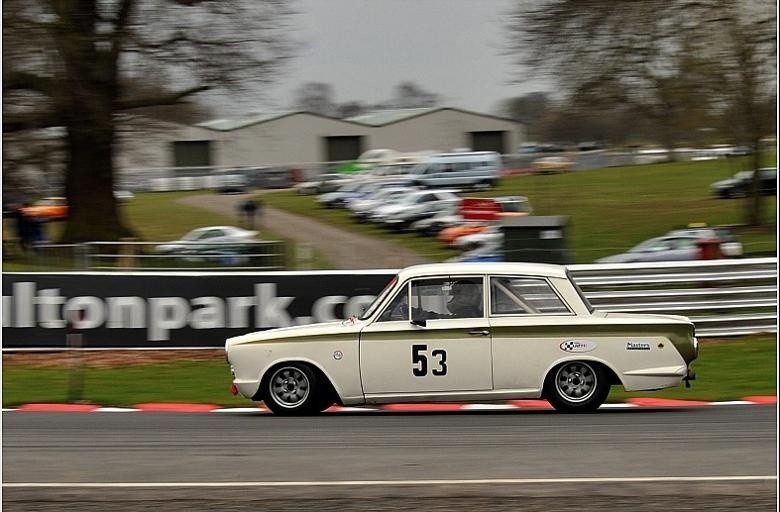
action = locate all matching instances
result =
[594,220,743,271]
[156,221,263,263]
[18,192,67,223]
[296,173,536,265]
[703,166,778,198]
[521,135,750,175]
[224,257,703,413]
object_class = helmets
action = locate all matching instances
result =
[447,281,481,313]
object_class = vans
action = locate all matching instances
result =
[414,151,505,191]
